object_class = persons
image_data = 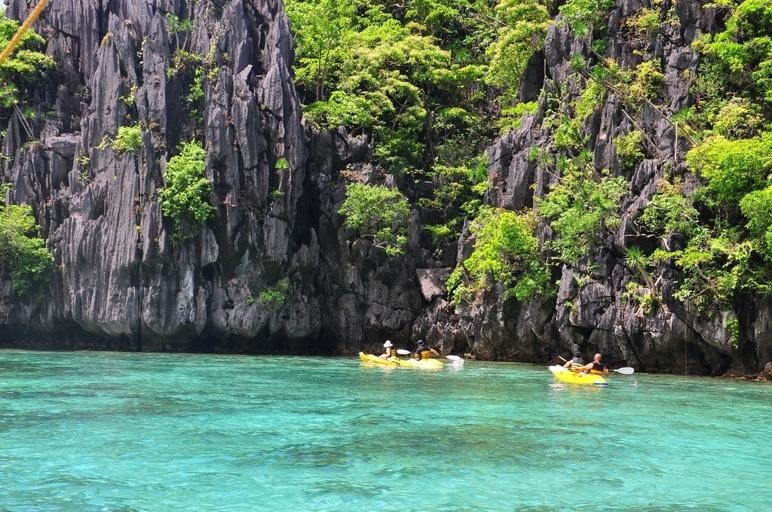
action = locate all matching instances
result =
[414,339,440,361]
[563,352,585,368]
[378,340,396,359]
[576,353,610,375]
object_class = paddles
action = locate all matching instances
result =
[440,354,460,362]
[397,349,410,356]
[608,367,634,374]
[558,356,567,362]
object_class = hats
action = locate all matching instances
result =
[416,340,424,345]
[574,352,582,357]
[383,340,393,348]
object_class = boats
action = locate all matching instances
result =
[359,352,414,370]
[548,364,608,389]
[407,357,444,370]
